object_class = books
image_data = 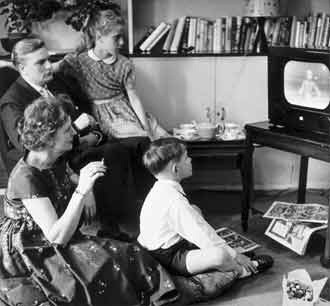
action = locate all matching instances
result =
[162,14,330,54]
[137,16,169,52]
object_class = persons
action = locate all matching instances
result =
[52,9,172,142]
[137,139,273,278]
[0,93,100,306]
[298,68,323,107]
[0,39,151,245]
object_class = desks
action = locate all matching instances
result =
[240,121,330,268]
[187,141,254,207]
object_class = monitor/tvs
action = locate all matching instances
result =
[267,48,330,138]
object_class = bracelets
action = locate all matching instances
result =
[73,189,88,199]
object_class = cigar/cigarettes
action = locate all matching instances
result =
[95,122,101,125]
[101,159,105,166]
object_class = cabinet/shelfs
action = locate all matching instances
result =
[131,3,330,58]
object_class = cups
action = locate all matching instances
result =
[197,122,225,138]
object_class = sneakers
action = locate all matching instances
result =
[250,254,274,274]
